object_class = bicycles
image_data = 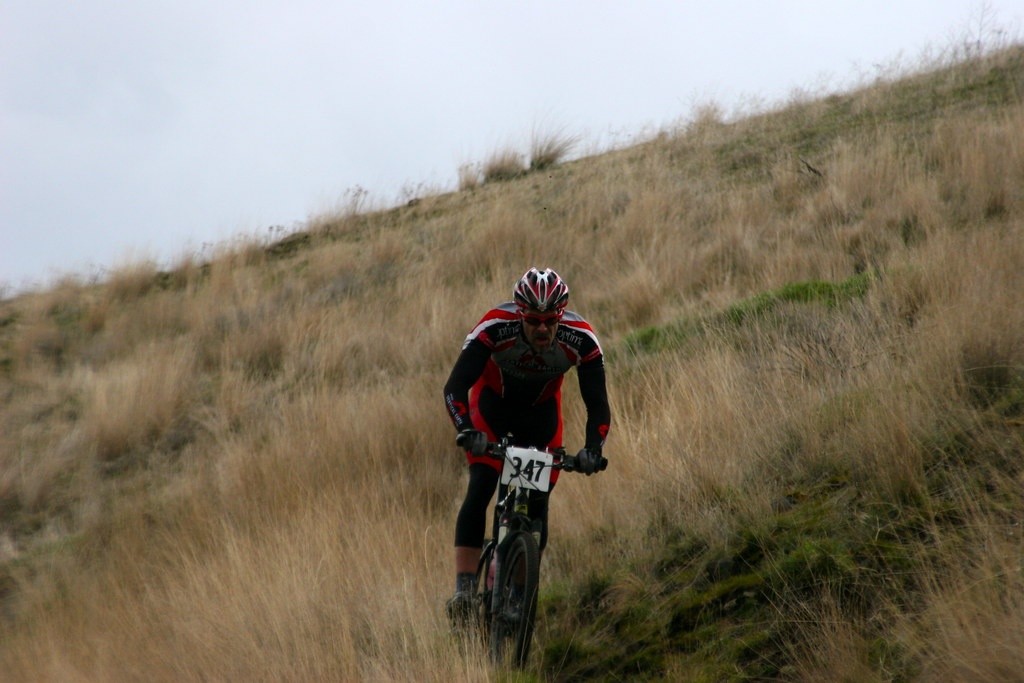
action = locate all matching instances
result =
[451,434,609,676]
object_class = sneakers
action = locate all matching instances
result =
[504,599,523,637]
[444,591,480,625]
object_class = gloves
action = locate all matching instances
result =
[573,445,602,476]
[456,428,488,457]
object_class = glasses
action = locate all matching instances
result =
[519,308,566,326]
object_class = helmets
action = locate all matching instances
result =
[514,266,569,314]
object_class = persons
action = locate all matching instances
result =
[442,268,612,619]
